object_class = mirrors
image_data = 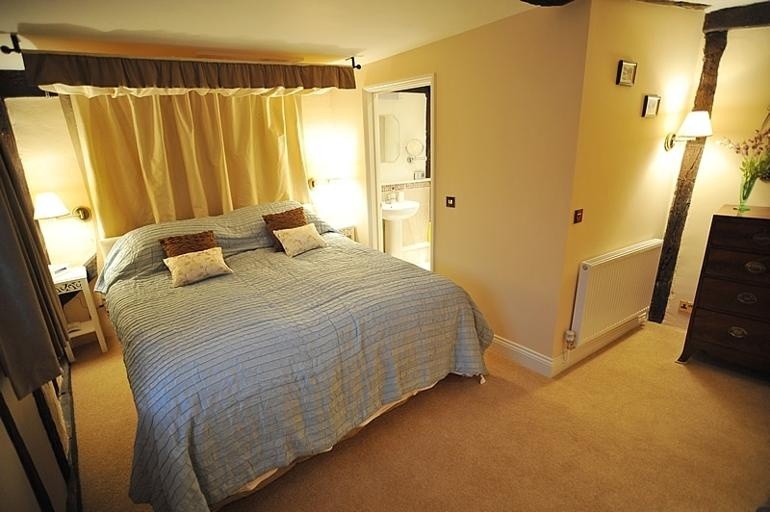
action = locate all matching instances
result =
[377,113,402,163]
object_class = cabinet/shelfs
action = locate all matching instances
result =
[673,207,769,380]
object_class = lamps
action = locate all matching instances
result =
[33,191,92,223]
[663,110,714,153]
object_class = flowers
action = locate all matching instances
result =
[721,103,770,200]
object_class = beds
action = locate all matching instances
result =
[99,199,466,512]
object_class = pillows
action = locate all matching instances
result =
[157,204,333,288]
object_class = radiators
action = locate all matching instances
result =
[564,237,664,348]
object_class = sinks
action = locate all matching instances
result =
[382,200,420,219]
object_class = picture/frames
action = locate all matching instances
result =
[640,94,662,118]
[615,59,639,87]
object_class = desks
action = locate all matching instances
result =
[47,265,109,364]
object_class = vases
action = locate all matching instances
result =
[733,177,758,213]
